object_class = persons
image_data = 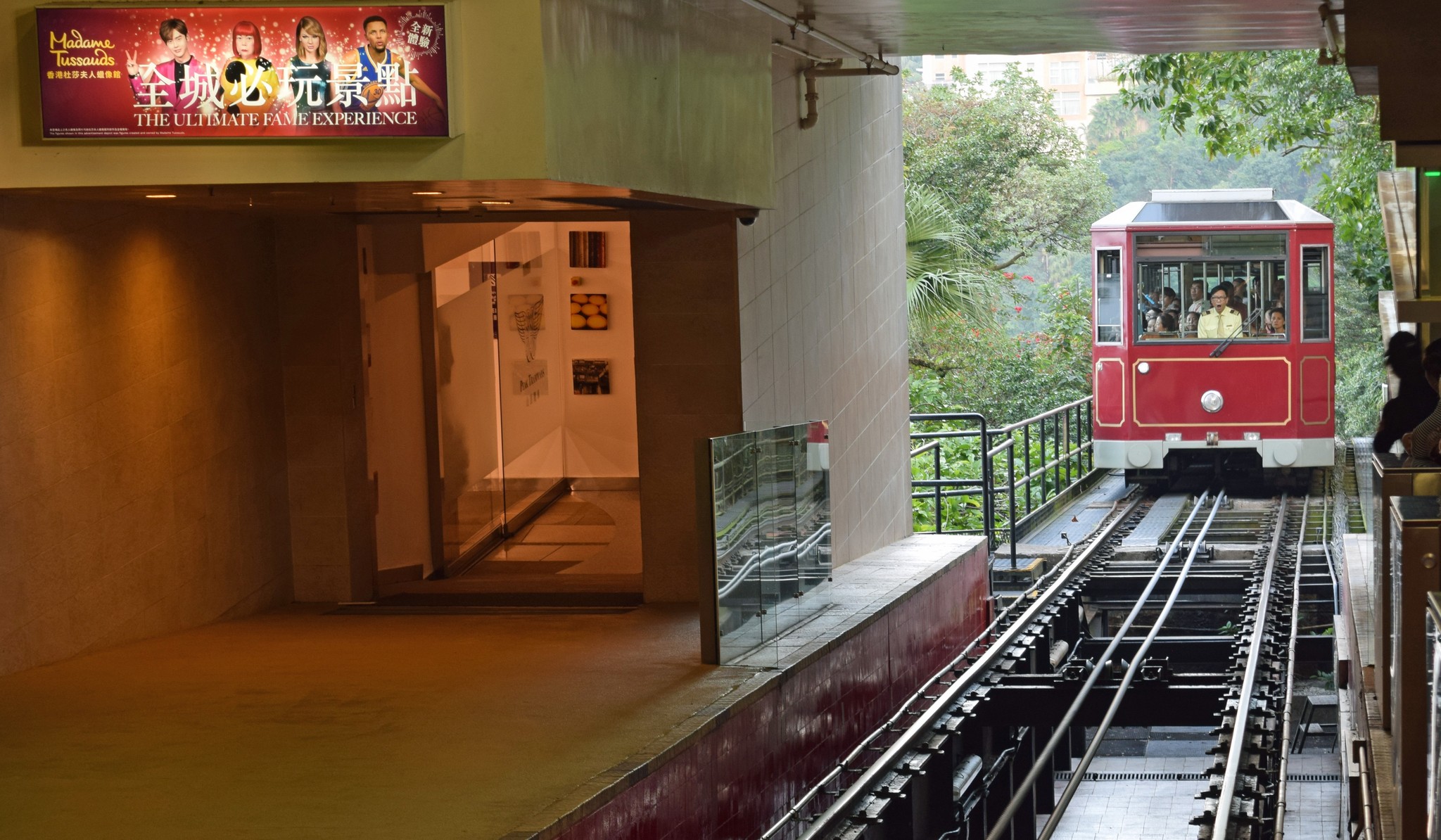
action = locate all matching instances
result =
[1140,272,1286,341]
[257,15,346,129]
[1370,328,1438,459]
[217,21,279,126]
[333,15,446,121]
[1196,286,1244,339]
[126,17,224,123]
[1401,336,1441,460]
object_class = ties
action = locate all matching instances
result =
[1217,315,1224,338]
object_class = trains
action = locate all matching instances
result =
[1088,187,1335,489]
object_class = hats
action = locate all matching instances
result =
[1384,330,1417,358]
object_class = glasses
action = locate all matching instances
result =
[1146,312,1153,319]
[1190,286,1202,290]
[1212,296,1226,301]
